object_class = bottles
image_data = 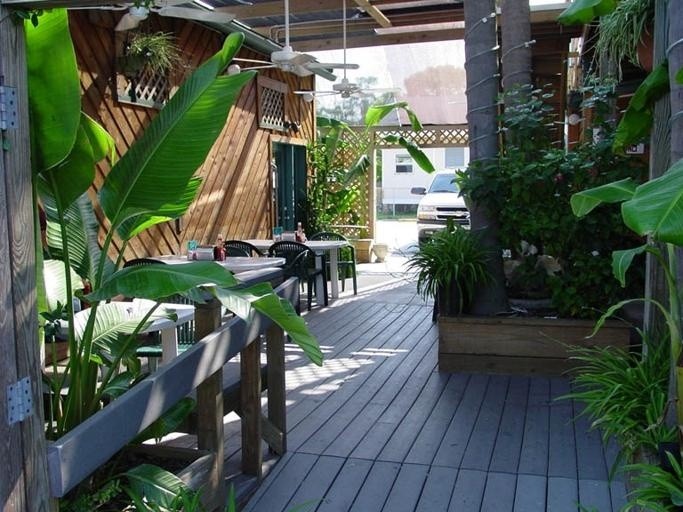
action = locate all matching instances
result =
[296,222,305,243]
[216,233,225,261]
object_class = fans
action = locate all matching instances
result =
[225,0,402,101]
[67,2,238,33]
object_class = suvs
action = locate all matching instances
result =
[411,167,471,252]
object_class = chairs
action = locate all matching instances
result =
[122,232,356,348]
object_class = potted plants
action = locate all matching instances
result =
[503,237,561,313]
[399,215,501,323]
